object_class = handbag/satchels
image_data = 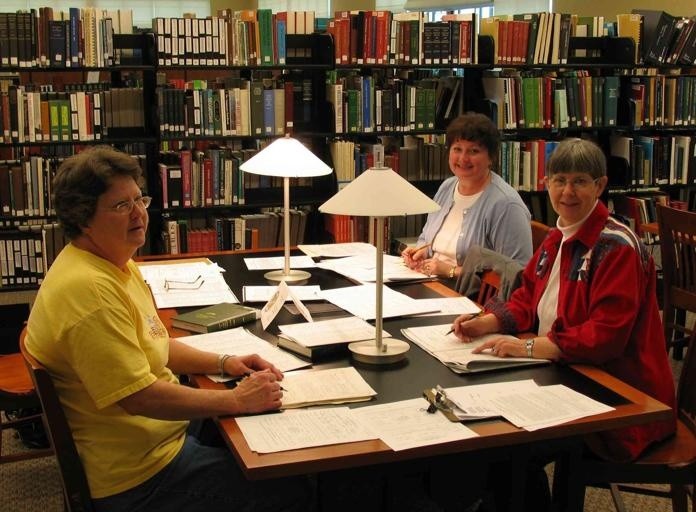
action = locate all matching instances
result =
[4,410,50,449]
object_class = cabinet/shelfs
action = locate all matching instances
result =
[2,55,695,306]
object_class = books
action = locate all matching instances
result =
[170,302,256,333]
[277,316,392,360]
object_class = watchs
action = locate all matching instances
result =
[525,338,534,358]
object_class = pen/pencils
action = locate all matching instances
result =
[244,372,288,392]
[445,310,483,336]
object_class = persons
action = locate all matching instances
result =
[24,144,297,512]
[450,137,678,512]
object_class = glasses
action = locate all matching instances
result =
[163,275,205,292]
[95,195,153,216]
[550,175,599,190]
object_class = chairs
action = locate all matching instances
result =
[0,202,696,512]
